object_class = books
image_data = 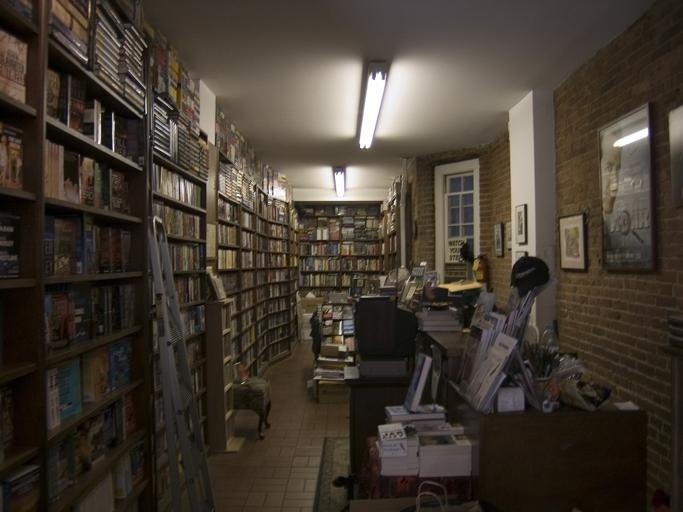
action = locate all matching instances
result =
[314,304,360,381]
[462,290,534,412]
[2,5,148,512]
[367,353,472,477]
[416,311,459,331]
[143,20,208,497]
[215,106,294,379]
[295,207,397,300]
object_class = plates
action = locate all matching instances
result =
[423,302,453,311]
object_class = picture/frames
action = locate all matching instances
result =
[493,221,504,259]
[558,212,588,272]
[504,220,512,251]
[513,203,527,245]
[596,100,659,275]
[515,251,527,262]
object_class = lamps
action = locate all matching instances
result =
[329,166,346,200]
[356,60,391,153]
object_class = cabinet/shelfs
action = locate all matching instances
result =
[344,293,651,510]
[216,148,239,384]
[3,2,155,511]
[239,175,290,378]
[290,179,406,301]
[152,90,207,511]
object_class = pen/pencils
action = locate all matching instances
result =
[523,340,559,380]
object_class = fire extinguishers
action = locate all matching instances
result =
[472,254,490,289]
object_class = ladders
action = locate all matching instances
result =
[148,215,216,512]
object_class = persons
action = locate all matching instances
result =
[599,122,624,253]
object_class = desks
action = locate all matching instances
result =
[313,299,355,404]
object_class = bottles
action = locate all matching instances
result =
[538,323,560,359]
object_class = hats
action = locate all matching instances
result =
[460,243,474,264]
[512,257,549,297]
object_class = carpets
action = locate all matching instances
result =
[310,435,350,511]
